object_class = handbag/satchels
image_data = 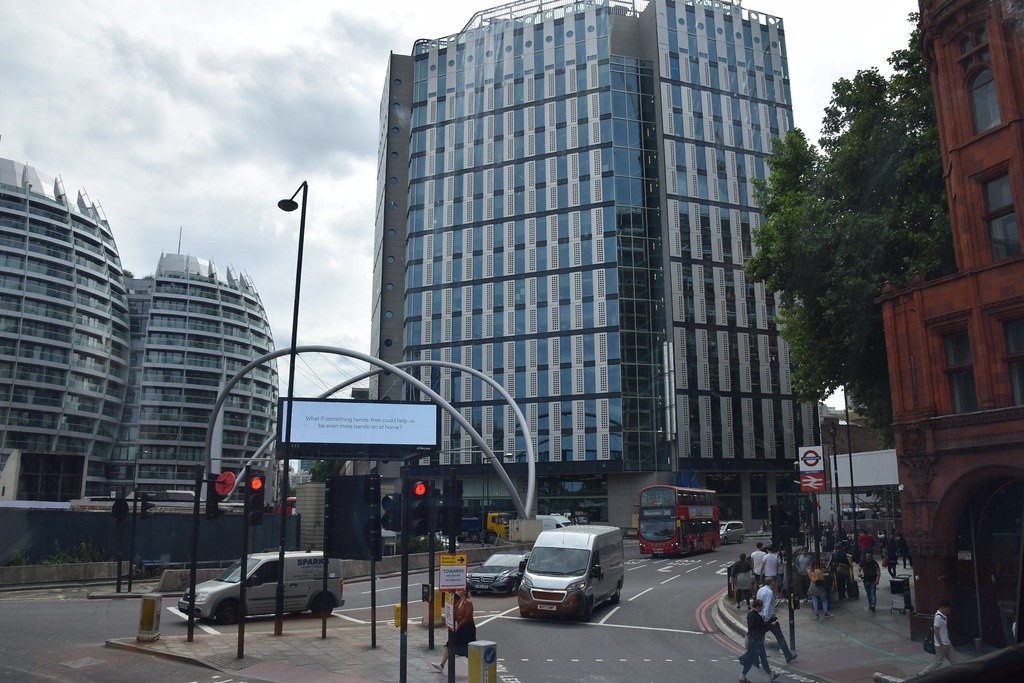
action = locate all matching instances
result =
[837,563,851,575]
[882,557,888,567]
[923,628,936,654]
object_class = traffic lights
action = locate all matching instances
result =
[141,492,156,519]
[205,473,228,519]
[407,480,430,535]
[381,493,401,531]
[248,474,265,525]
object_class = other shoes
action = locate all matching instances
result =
[748,606,752,609]
[824,613,833,617]
[868,604,876,610]
[737,605,741,608]
[431,662,444,672]
[786,653,797,662]
[815,613,819,618]
[739,678,753,683]
[770,673,780,680]
[803,597,808,602]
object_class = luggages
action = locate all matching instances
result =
[846,566,860,599]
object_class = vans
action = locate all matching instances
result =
[535,515,573,531]
[519,525,624,622]
[178,550,346,624]
[719,521,745,545]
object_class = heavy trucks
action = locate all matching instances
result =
[457,512,514,544]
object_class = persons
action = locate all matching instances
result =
[431,583,476,670]
[738,599,780,683]
[917,600,957,675]
[756,577,798,666]
[731,519,912,617]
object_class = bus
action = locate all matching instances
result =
[842,508,871,520]
[638,485,721,556]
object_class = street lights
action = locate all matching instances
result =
[482,453,514,547]
[128,450,148,593]
[274,182,307,635]
[830,421,842,534]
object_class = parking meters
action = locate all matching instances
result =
[788,592,800,651]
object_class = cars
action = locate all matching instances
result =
[276,496,296,516]
[466,550,533,596]
